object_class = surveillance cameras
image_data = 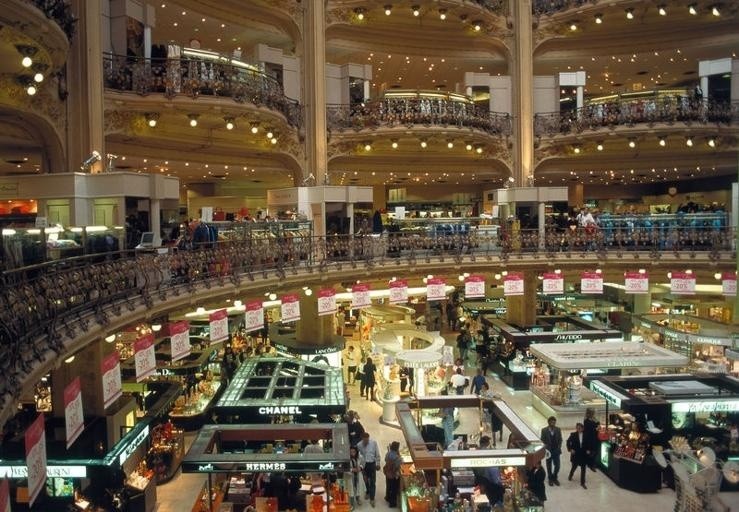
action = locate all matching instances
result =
[503,177,515,189]
[79,150,102,172]
[106,153,119,173]
[527,175,535,187]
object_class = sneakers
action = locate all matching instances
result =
[351,493,397,507]
[549,477,588,489]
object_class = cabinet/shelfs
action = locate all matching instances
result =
[31,319,739,512]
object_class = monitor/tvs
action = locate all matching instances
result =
[141,231,162,248]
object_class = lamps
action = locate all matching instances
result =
[419,137,429,149]
[248,121,261,135]
[593,13,605,24]
[356,8,367,21]
[446,139,455,149]
[685,136,695,148]
[16,44,46,95]
[383,6,394,16]
[363,143,374,151]
[502,176,516,189]
[624,8,635,20]
[705,136,717,149]
[188,114,199,127]
[711,7,721,17]
[572,144,581,154]
[596,140,605,151]
[301,173,315,187]
[438,7,448,20]
[474,22,482,30]
[465,141,473,151]
[658,6,668,16]
[145,114,161,128]
[265,127,280,144]
[570,22,577,31]
[411,6,421,16]
[627,137,638,149]
[80,150,102,172]
[196,286,312,316]
[475,144,484,154]
[223,117,237,131]
[422,268,723,284]
[106,153,118,172]
[688,6,697,16]
[390,138,400,149]
[659,136,667,148]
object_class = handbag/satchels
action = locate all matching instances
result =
[354,371,365,380]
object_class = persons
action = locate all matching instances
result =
[541,416,563,486]
[439,390,503,510]
[167,212,189,242]
[566,423,592,489]
[583,407,602,472]
[574,207,597,250]
[415,302,489,396]
[591,208,601,225]
[344,346,376,402]
[222,409,403,510]
[223,343,270,379]
[529,462,547,506]
